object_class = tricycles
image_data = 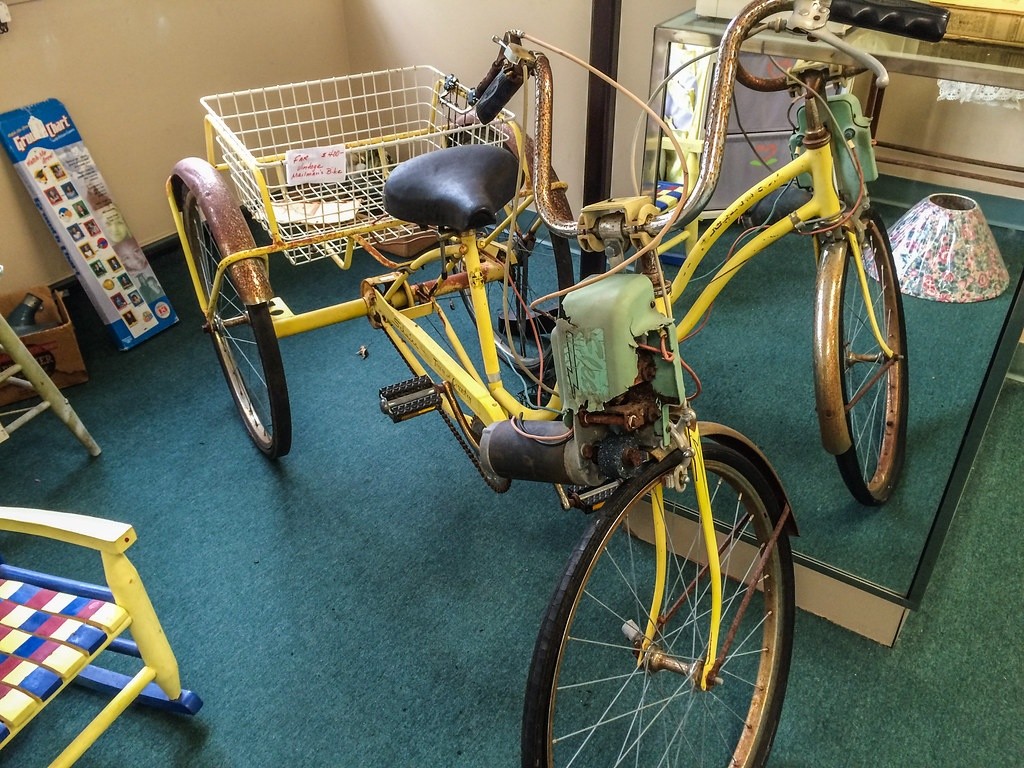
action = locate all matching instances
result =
[670,56,910,510]
[163,0,951,768]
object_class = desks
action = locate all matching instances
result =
[640,8,1024,209]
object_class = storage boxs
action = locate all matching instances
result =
[0,286,88,407]
[0,97,179,351]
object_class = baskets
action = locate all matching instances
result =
[200,63,516,266]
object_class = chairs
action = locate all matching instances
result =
[0,506,203,768]
